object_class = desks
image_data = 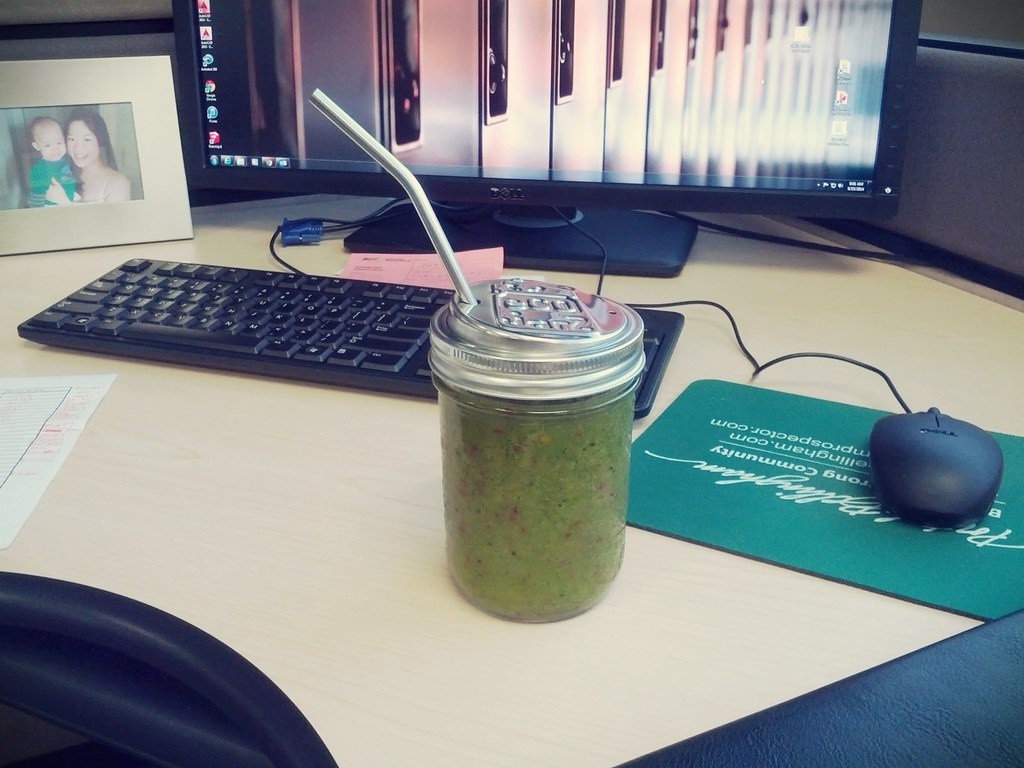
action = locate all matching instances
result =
[0,201,1024,768]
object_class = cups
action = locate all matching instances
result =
[429,279,645,620]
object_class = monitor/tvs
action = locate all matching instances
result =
[173,0,925,278]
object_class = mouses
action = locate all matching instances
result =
[871,408,1004,530]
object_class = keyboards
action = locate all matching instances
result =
[19,256,685,419]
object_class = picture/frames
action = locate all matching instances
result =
[0,54,194,257]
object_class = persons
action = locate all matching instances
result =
[25,109,131,210]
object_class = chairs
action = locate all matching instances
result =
[0,569,337,768]
